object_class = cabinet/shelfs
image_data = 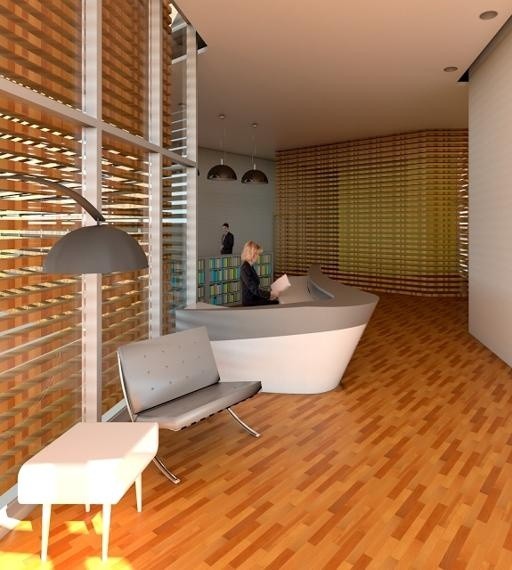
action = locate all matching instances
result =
[170,253,274,326]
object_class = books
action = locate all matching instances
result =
[210,295,223,305]
[198,272,205,284]
[208,258,219,268]
[198,259,204,271]
[209,283,222,295]
[198,286,206,298]
[261,287,270,291]
[223,281,241,293]
[224,292,241,304]
[223,257,240,267]
[209,269,222,281]
[253,264,272,276]
[222,268,240,281]
[271,273,291,293]
[259,277,271,288]
[254,254,272,264]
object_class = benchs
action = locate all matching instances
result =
[116,326,262,484]
[18,422,159,563]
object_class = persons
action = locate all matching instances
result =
[220,222,235,254]
[240,241,281,306]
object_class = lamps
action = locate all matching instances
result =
[0,172,149,274]
[241,123,268,184]
[207,115,237,181]
